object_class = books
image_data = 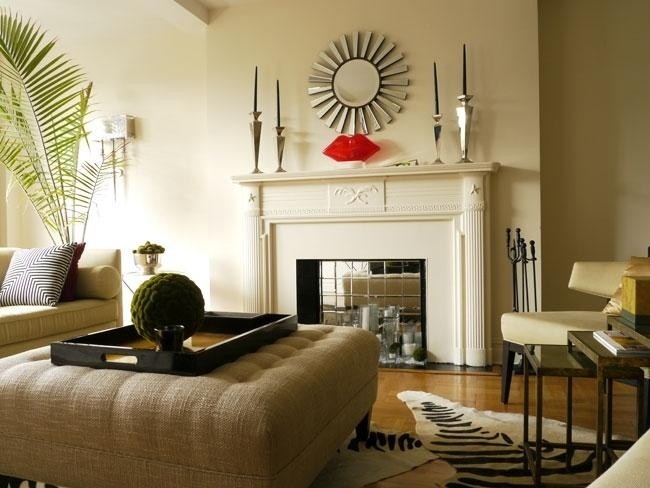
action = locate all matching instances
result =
[592,330,650,358]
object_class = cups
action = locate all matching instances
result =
[402,331,416,359]
[155,326,183,352]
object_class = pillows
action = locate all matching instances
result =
[0,243,86,308]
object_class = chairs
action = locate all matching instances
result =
[500,260,627,406]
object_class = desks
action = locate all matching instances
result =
[523,330,650,486]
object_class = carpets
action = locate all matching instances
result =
[0,389,643,488]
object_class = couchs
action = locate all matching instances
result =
[0,243,123,361]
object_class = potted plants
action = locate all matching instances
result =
[132,241,165,276]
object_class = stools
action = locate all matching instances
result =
[0,324,385,488]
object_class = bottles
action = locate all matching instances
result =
[383,310,395,357]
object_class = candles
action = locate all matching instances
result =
[276,80,280,126]
[434,62,440,114]
[253,66,258,112]
[461,42,467,94]
